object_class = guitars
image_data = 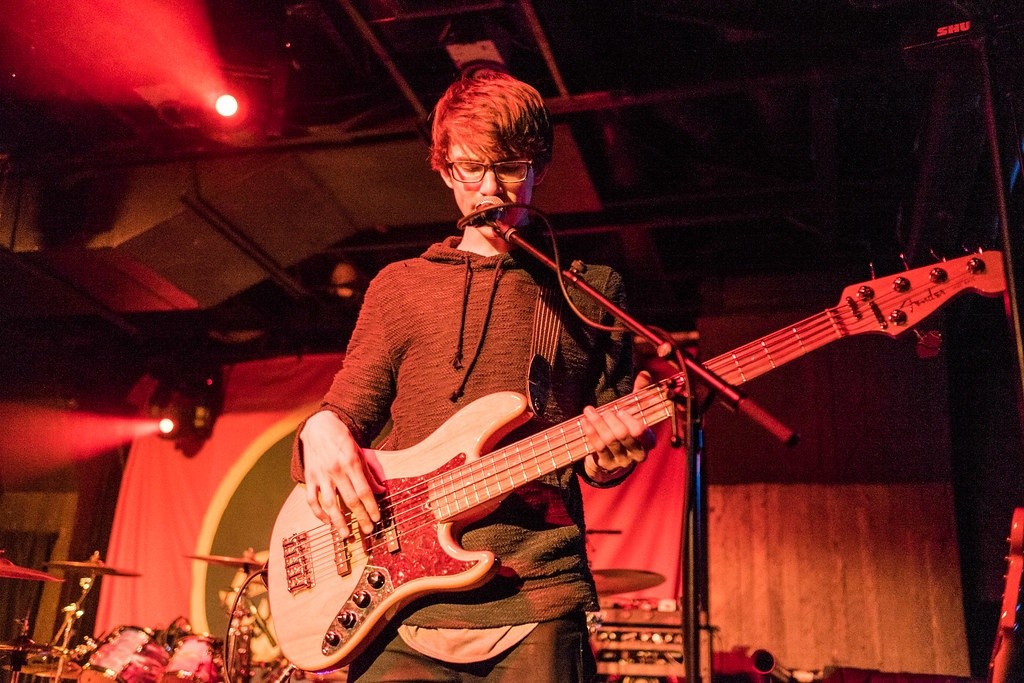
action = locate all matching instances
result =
[266,238,1008,675]
[986,505,1024,683]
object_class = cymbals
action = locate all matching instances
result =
[588,568,666,596]
[180,552,264,572]
[44,558,142,579]
[0,557,65,584]
[0,636,82,682]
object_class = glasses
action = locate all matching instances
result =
[444,154,534,184]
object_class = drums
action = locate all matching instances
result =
[78,617,225,683]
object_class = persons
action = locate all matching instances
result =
[292,66,659,683]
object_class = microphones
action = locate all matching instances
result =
[245,599,276,649]
[468,196,505,228]
